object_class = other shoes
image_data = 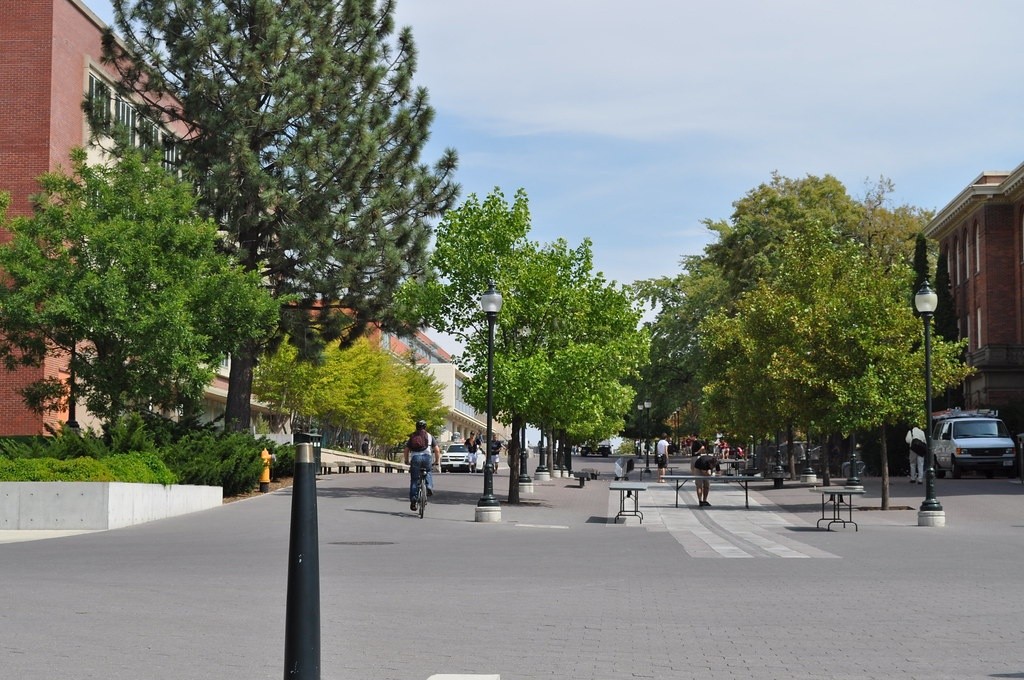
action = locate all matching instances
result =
[700,502,703,506]
[657,480,665,483]
[918,482,923,484]
[427,486,432,495]
[704,502,711,506]
[910,480,916,483]
[410,498,417,511]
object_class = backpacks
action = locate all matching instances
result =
[408,430,428,451]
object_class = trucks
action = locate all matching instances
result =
[581,431,613,458]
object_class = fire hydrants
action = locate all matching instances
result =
[258,447,278,492]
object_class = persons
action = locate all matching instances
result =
[681,438,687,455]
[657,433,669,483]
[686,436,692,455]
[713,438,730,459]
[691,433,705,483]
[694,455,721,506]
[905,422,927,484]
[404,420,440,511]
[505,433,521,467]
[464,432,481,473]
[491,435,501,474]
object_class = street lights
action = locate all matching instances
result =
[477,279,506,524]
[642,399,653,478]
[513,319,535,494]
[912,280,949,527]
[638,403,646,459]
[675,403,684,457]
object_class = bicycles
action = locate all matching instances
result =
[408,460,439,521]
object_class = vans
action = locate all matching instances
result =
[930,411,1018,478]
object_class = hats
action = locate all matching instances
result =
[470,432,475,435]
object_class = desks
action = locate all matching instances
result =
[610,481,647,524]
[634,466,678,483]
[808,487,867,531]
[661,474,764,508]
[725,458,749,476]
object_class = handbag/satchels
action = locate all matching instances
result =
[911,439,926,457]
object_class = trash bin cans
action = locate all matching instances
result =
[293,432,322,474]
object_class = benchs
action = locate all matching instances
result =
[321,459,410,475]
[574,467,600,488]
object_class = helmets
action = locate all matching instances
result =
[416,420,426,429]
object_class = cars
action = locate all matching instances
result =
[439,443,488,473]
